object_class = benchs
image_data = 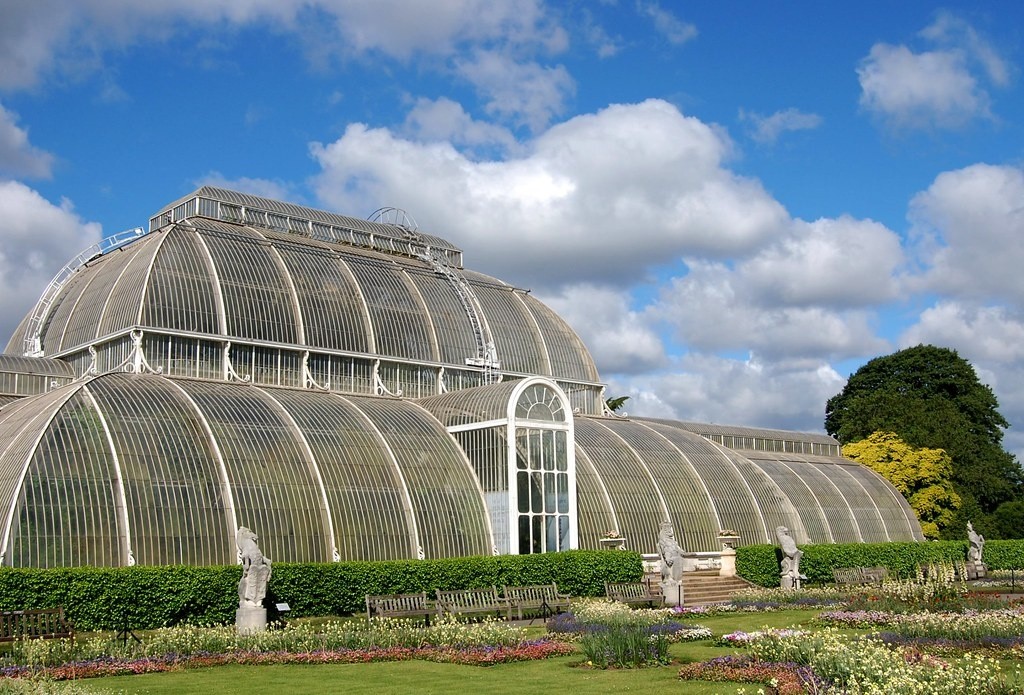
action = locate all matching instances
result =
[831,567,875,591]
[365,591,444,630]
[434,586,512,625]
[918,563,960,584]
[957,560,989,581]
[501,582,571,621]
[862,566,898,588]
[0,605,76,655]
[603,579,664,609]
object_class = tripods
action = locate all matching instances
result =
[113,620,141,647]
[529,594,555,625]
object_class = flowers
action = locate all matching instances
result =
[600,530,623,539]
[719,530,739,536]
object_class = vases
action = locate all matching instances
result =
[600,538,625,547]
[717,536,740,543]
